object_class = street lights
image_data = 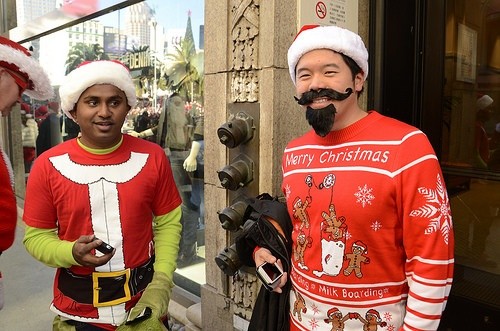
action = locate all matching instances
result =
[82,20,99,60]
[152,21,158,113]
[103,26,114,55]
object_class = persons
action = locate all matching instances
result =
[23,59,182,331]
[21,101,202,184]
[129,72,204,267]
[0,35,55,311]
[437,95,500,266]
[254,26,456,331]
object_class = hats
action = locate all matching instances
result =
[59,59,136,121]
[21,103,30,114]
[287,25,368,86]
[35,105,51,119]
[0,35,54,102]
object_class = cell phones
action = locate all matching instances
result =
[259,260,284,285]
[91,237,113,254]
